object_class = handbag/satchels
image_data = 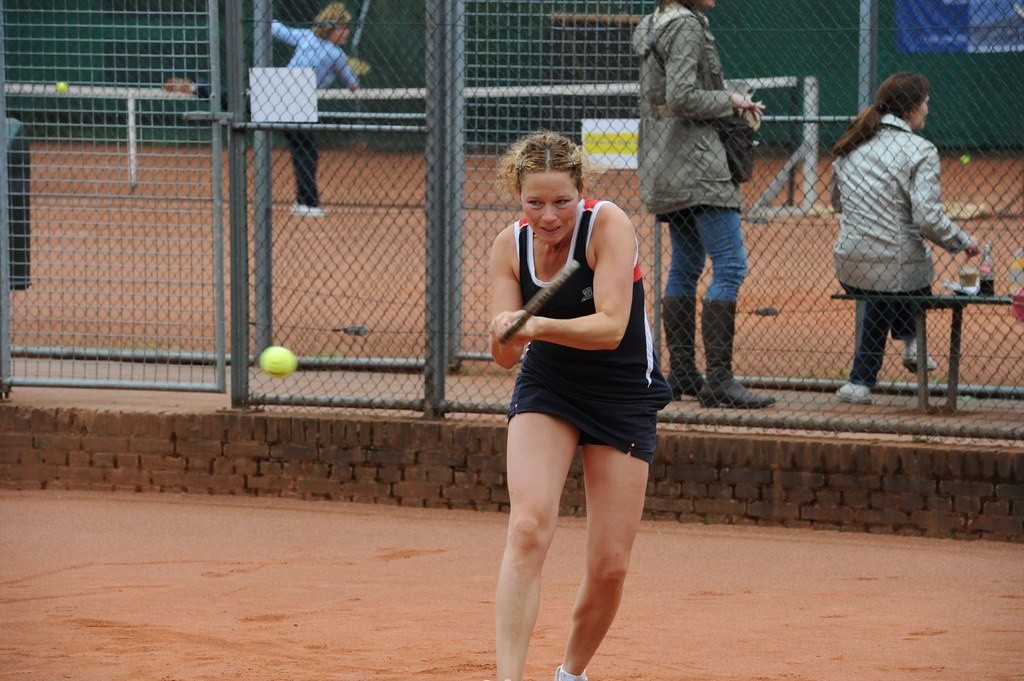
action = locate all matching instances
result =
[719,118,757,184]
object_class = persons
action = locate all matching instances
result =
[826,70,982,407]
[620,1,783,409]
[268,0,363,219]
[485,125,665,681]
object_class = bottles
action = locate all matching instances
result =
[978,244,994,296]
[1007,248,1024,299]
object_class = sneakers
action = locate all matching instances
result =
[903,338,938,373]
[835,380,872,404]
[289,200,326,218]
[162,77,195,94]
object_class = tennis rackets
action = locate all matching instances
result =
[496,260,584,347]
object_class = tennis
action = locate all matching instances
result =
[260,347,297,378]
[961,154,972,166]
[56,81,69,96]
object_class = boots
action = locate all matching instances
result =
[699,299,777,410]
[662,295,704,400]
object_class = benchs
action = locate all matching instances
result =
[836,287,1015,406]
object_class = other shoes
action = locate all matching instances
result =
[551,665,589,681]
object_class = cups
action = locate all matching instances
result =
[959,268,980,287]
